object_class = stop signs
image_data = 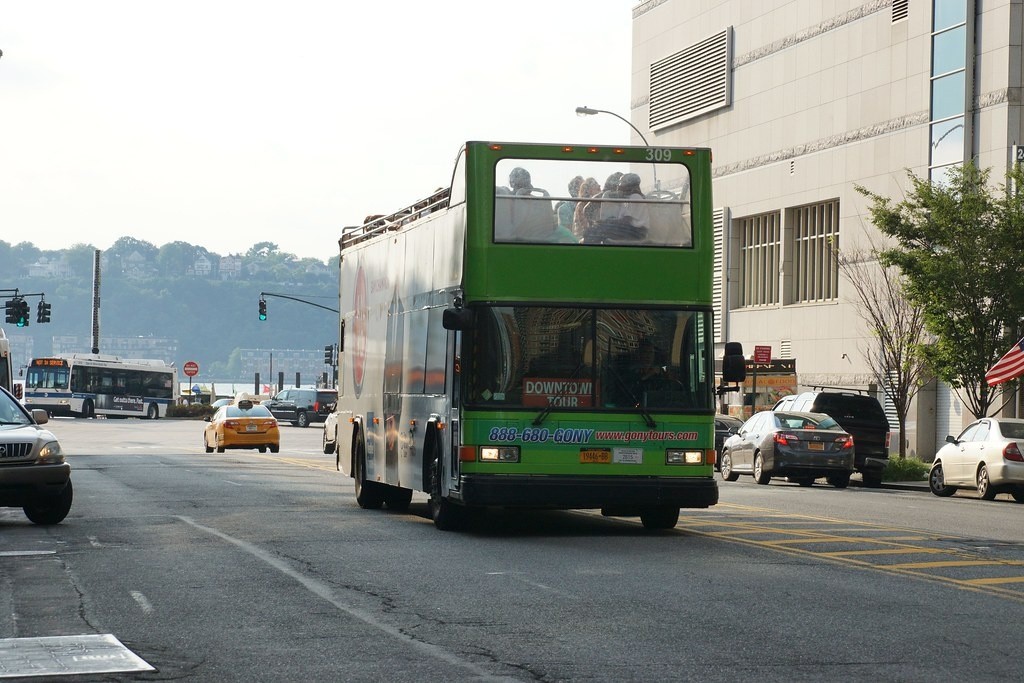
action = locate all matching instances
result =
[184,362,198,376]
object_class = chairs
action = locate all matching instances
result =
[802,418,818,426]
[646,190,688,245]
[512,187,554,242]
[495,186,516,241]
[600,190,649,245]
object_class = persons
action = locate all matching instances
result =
[558,175,586,230]
[571,178,602,238]
[582,171,623,226]
[495,168,535,195]
[63,373,76,389]
[583,173,650,243]
[552,201,566,214]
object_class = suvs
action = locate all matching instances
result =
[0,385,75,524]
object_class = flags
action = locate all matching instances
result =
[985,337,1024,386]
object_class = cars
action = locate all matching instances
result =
[202,399,280,453]
[213,398,234,422]
[928,417,1024,501]
[322,400,339,456]
[714,414,746,472]
[720,410,855,487]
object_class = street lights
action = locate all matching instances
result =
[575,107,659,193]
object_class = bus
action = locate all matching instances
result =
[0,327,15,422]
[335,139,749,531]
[19,352,180,420]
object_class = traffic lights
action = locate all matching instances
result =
[13,298,27,327]
[5,299,16,325]
[23,306,30,326]
[37,303,52,323]
[324,345,333,365]
[258,300,267,321]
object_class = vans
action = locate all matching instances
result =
[260,388,339,427]
[767,382,891,487]
